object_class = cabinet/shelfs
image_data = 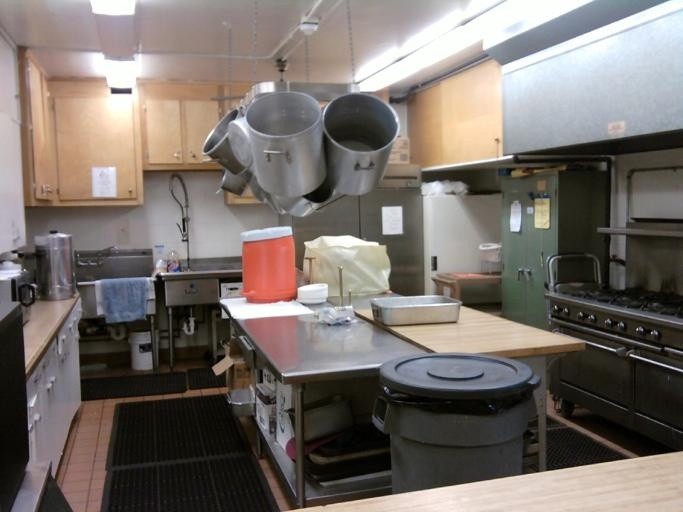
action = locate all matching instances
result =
[138,80,223,173]
[26,298,82,480]
[219,84,270,204]
[407,56,501,170]
[20,47,146,208]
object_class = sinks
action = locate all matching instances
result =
[76,262,157,320]
[162,272,219,307]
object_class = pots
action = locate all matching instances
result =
[201,91,400,217]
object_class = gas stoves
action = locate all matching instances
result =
[550,283,683,351]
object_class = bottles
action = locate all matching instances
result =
[154,245,180,272]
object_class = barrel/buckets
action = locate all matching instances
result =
[371,351,542,497]
[127,324,161,371]
[241,225,297,303]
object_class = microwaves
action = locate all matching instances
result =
[1,268,34,325]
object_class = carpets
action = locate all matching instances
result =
[530,411,679,473]
[81,365,227,402]
[98,393,280,512]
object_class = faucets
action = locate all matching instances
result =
[168,173,191,270]
[95,245,119,268]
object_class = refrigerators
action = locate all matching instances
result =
[278,163,425,295]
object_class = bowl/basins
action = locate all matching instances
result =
[296,283,328,305]
